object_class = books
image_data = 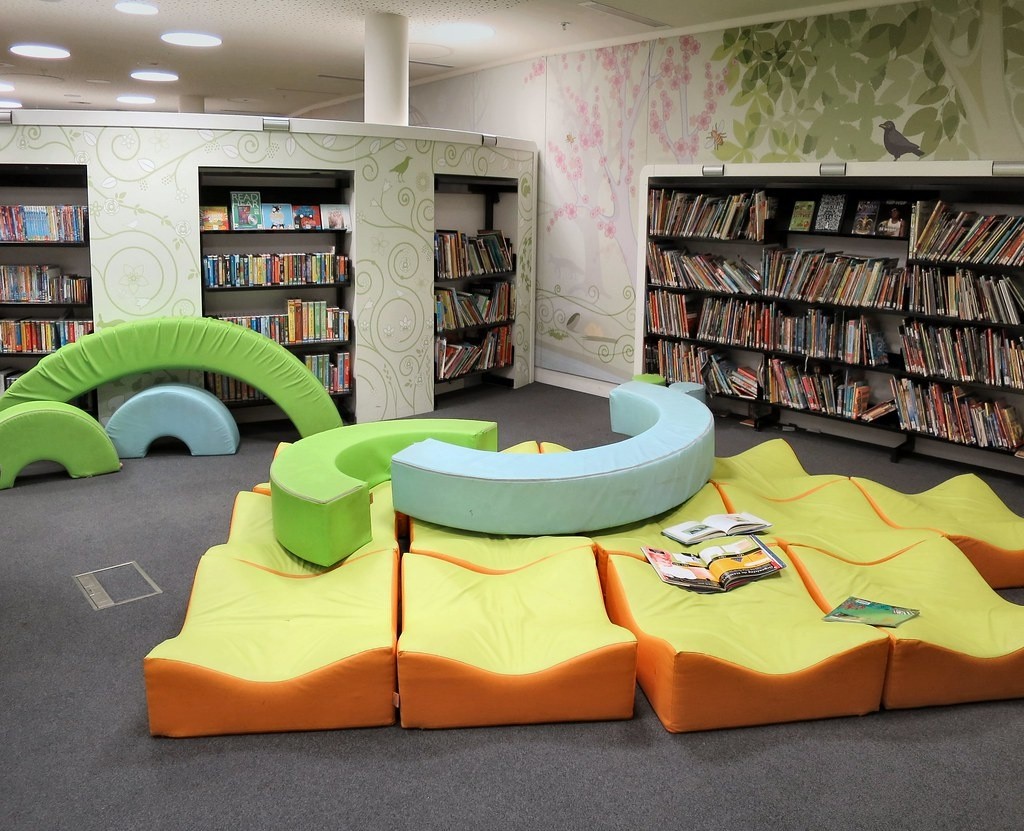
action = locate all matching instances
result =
[434,281,516,332]
[821,596,920,627]
[0,205,87,242]
[435,325,512,380]
[218,297,350,344]
[0,369,24,392]
[434,229,513,279]
[661,512,772,545]
[640,533,787,594]
[645,188,1024,459]
[0,318,93,353]
[208,353,350,402]
[200,192,351,230]
[0,265,89,303]
[203,245,348,288]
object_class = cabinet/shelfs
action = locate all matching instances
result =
[198,166,355,406]
[0,163,98,419]
[640,176,1023,482]
[434,173,516,384]
